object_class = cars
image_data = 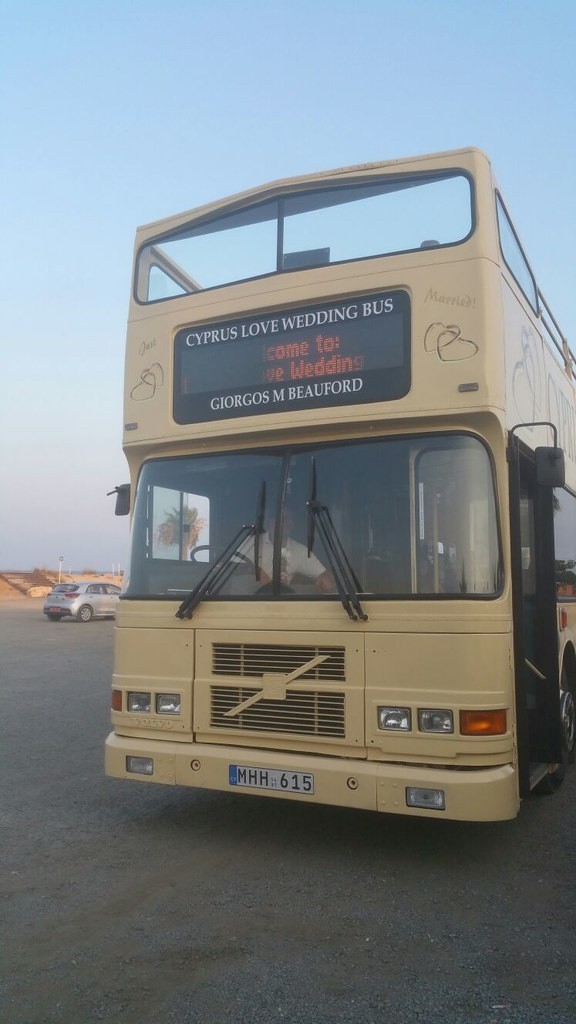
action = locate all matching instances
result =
[43,582,121,623]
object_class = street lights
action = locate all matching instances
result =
[58,555,64,584]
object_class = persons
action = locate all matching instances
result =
[227,508,333,594]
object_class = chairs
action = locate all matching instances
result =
[92,587,99,593]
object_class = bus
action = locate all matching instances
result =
[104,148,576,821]
